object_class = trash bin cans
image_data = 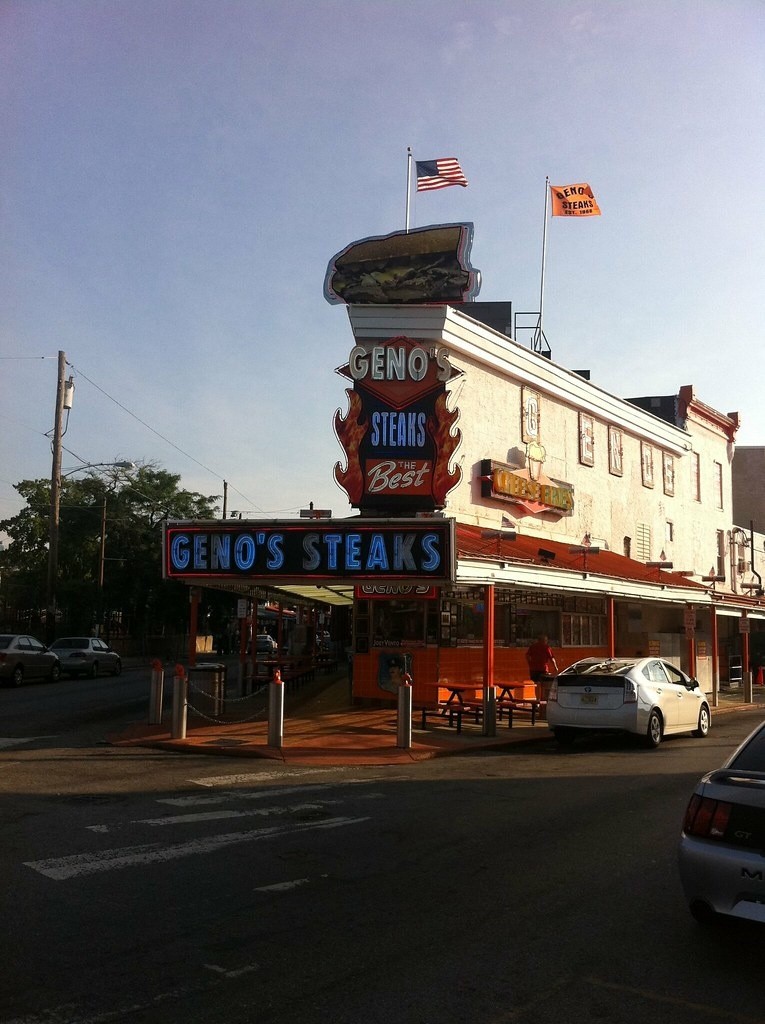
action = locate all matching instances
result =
[189,663,228,718]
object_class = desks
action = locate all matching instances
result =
[475,679,538,728]
[425,681,484,733]
[256,660,293,676]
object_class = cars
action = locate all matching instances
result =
[17,606,133,636]
[48,636,122,681]
[315,630,331,648]
[678,719,765,932]
[0,633,62,689]
[247,635,278,655]
[546,656,712,750]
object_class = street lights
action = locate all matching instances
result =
[45,460,132,642]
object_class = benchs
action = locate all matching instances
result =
[413,696,547,712]
[245,659,340,682]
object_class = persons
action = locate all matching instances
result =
[525,634,558,700]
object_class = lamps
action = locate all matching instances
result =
[645,561,674,581]
[538,548,557,563]
[741,583,763,597]
[702,576,725,590]
[568,545,600,572]
[480,529,517,557]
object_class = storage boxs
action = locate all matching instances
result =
[729,682,739,689]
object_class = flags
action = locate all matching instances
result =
[415,158,469,192]
[551,183,602,217]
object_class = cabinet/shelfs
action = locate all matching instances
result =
[729,655,743,686]
